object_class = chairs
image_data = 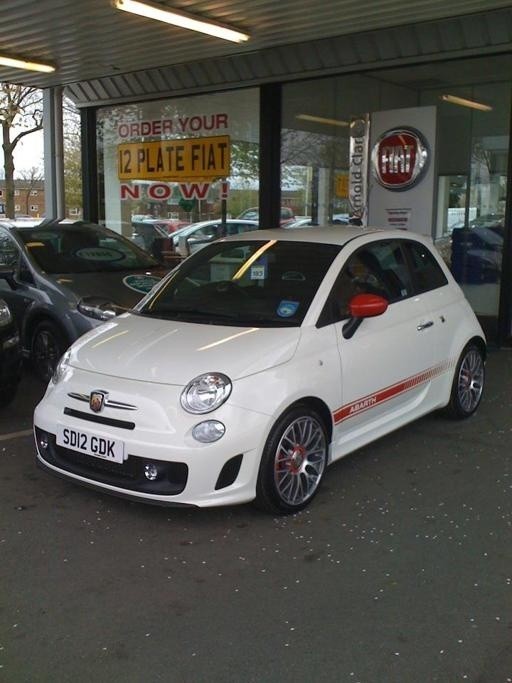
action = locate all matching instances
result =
[311,259,359,316]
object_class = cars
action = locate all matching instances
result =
[131,206,348,256]
[0,218,173,383]
[34,226,487,514]
